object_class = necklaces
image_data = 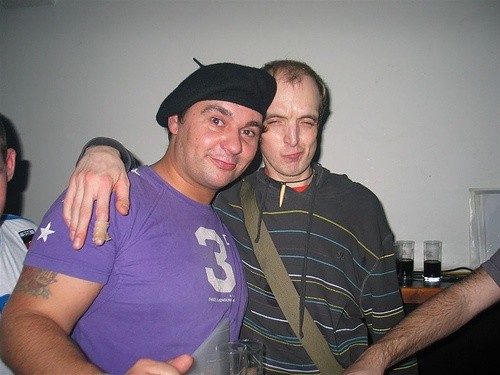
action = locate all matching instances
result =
[273,170,315,207]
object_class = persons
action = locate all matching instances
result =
[1,124,40,375]
[341,244,500,374]
[1,56,277,375]
[61,59,406,375]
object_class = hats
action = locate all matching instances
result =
[156,57,277,128]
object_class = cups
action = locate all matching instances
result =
[395,241,414,287]
[232,340,264,375]
[424,240,443,283]
[201,340,248,375]
